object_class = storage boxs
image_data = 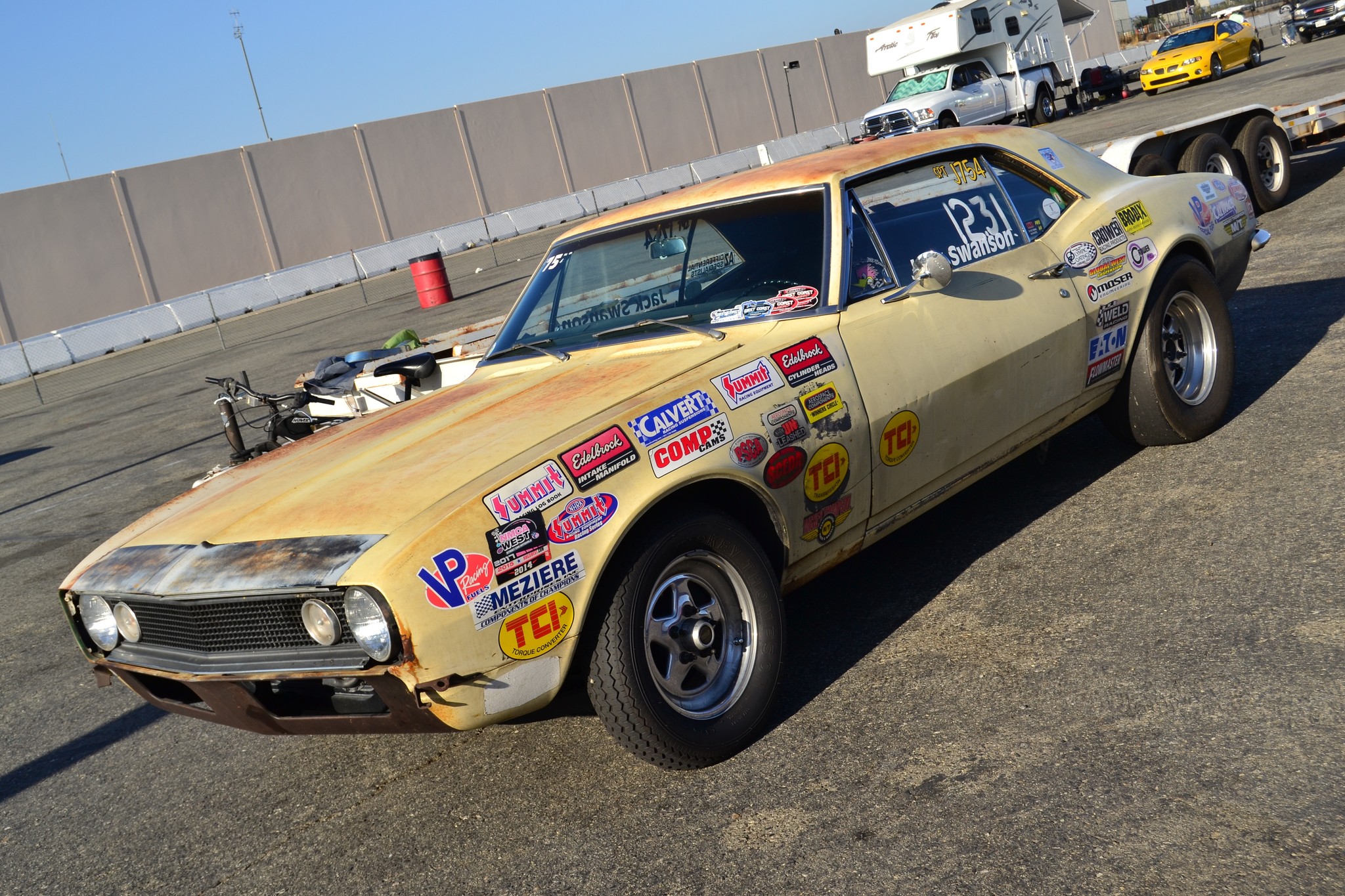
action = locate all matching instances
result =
[1098,95,1106,101]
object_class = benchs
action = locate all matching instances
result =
[853,193,1052,269]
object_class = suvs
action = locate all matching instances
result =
[1293,0,1345,43]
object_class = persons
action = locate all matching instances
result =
[1219,10,1249,25]
[1279,0,1297,45]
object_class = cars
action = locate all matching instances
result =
[1140,18,1263,98]
[58,123,1270,773]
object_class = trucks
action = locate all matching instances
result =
[859,0,1099,144]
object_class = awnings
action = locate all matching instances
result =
[1210,4,1252,17]
[1056,0,1098,27]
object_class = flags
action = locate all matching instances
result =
[1186,3,1194,15]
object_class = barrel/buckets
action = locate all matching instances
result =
[407,251,454,309]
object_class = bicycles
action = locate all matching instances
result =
[190,352,435,491]
